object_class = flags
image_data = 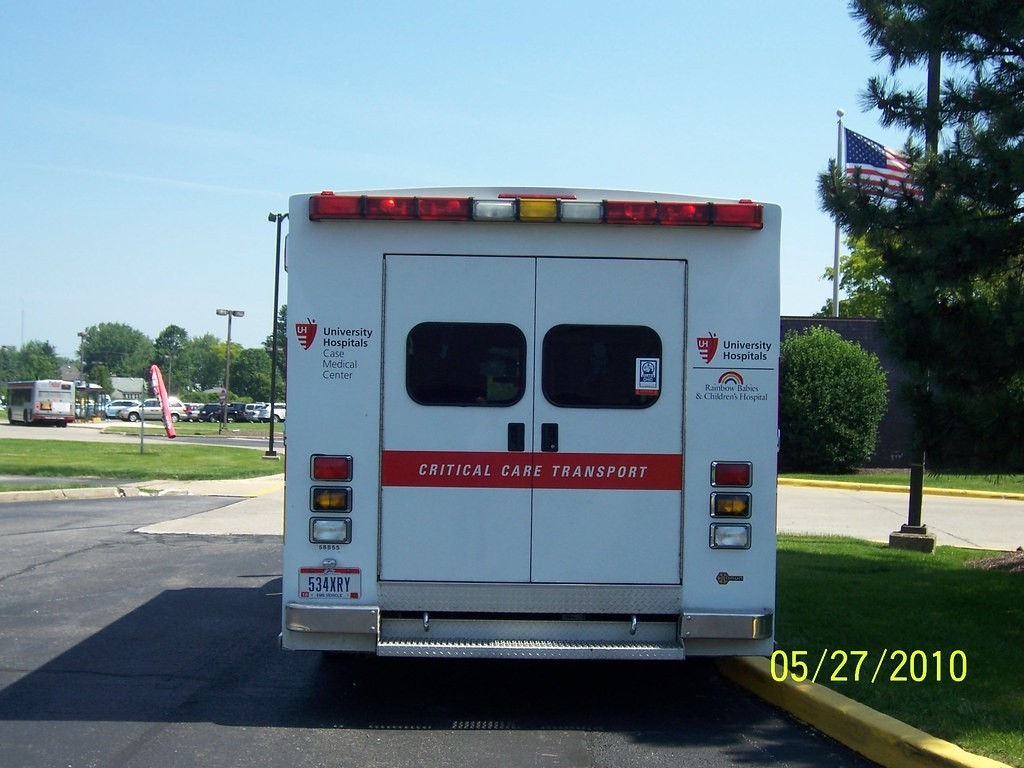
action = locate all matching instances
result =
[843,127,924,199]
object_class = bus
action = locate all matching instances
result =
[6,379,76,428]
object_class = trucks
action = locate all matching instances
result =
[282,186,782,660]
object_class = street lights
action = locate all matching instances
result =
[216,309,244,428]
[78,332,87,418]
[266,212,289,457]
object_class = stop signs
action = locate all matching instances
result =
[219,390,226,404]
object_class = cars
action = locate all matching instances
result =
[184,402,205,422]
[117,398,188,423]
[198,403,230,423]
[214,402,265,422]
[89,400,142,419]
[258,403,286,422]
